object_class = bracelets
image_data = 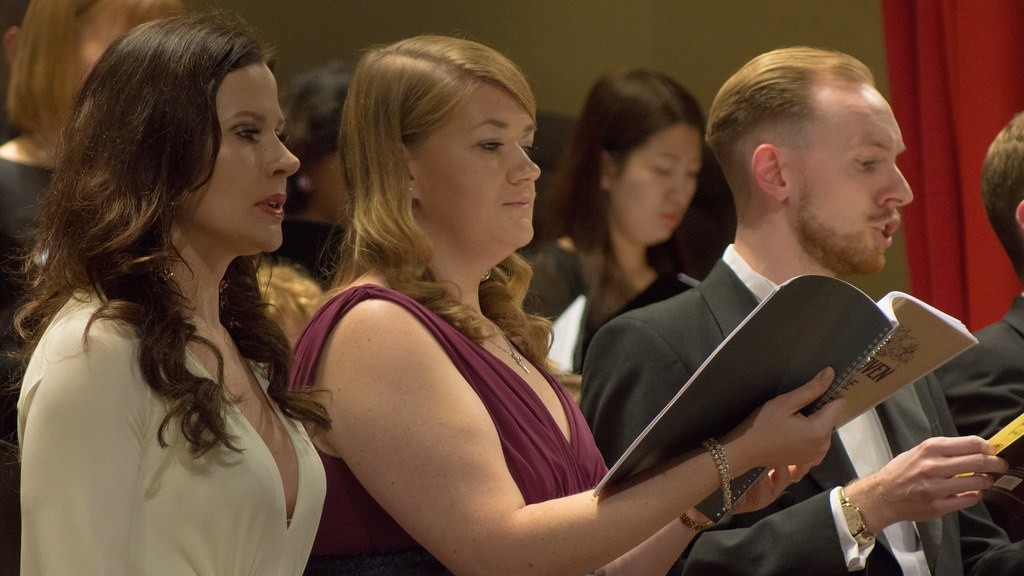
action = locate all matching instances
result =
[701,437,732,516]
[680,513,712,530]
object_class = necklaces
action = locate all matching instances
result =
[481,317,530,373]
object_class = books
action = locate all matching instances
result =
[954,412,1024,502]
[593,274,979,525]
[549,295,589,374]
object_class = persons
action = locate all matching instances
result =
[0,0,326,576]
[261,57,737,376]
[281,34,849,575]
[578,46,1024,576]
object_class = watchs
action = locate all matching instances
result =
[838,477,877,546]
